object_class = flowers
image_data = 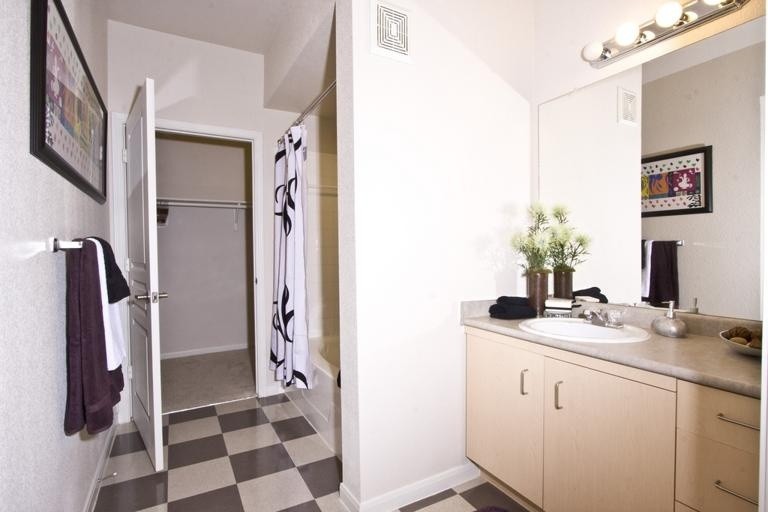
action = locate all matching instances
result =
[509,201,556,277]
[546,203,591,271]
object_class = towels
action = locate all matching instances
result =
[489,295,537,320]
[62,235,134,438]
[573,286,608,303]
[641,238,680,309]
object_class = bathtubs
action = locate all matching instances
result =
[282,333,343,462]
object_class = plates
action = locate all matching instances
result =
[718,330,761,357]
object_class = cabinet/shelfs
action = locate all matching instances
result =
[463,324,677,512]
[674,379,760,509]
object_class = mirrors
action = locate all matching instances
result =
[535,13,767,325]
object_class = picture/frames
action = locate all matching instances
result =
[30,0,110,204]
[641,144,714,217]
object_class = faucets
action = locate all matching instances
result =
[582,310,608,325]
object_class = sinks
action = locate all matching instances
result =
[518,316,651,343]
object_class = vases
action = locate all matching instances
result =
[553,271,573,301]
[526,273,548,316]
